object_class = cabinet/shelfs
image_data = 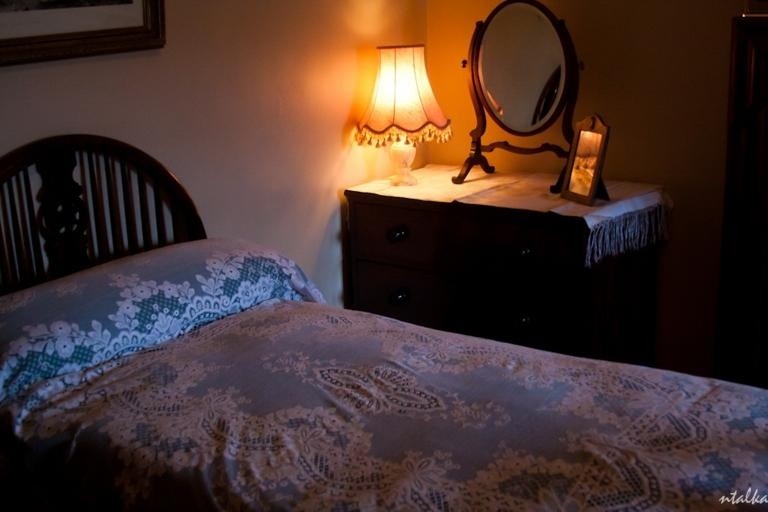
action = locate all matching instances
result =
[345,171,673,363]
[712,15,768,391]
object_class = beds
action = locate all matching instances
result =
[0,135,767,511]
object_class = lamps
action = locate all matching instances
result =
[345,43,453,185]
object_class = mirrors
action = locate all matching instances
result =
[452,0,580,195]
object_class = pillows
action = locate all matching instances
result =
[0,234,327,414]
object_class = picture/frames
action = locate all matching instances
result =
[0,0,167,64]
[561,114,611,206]
